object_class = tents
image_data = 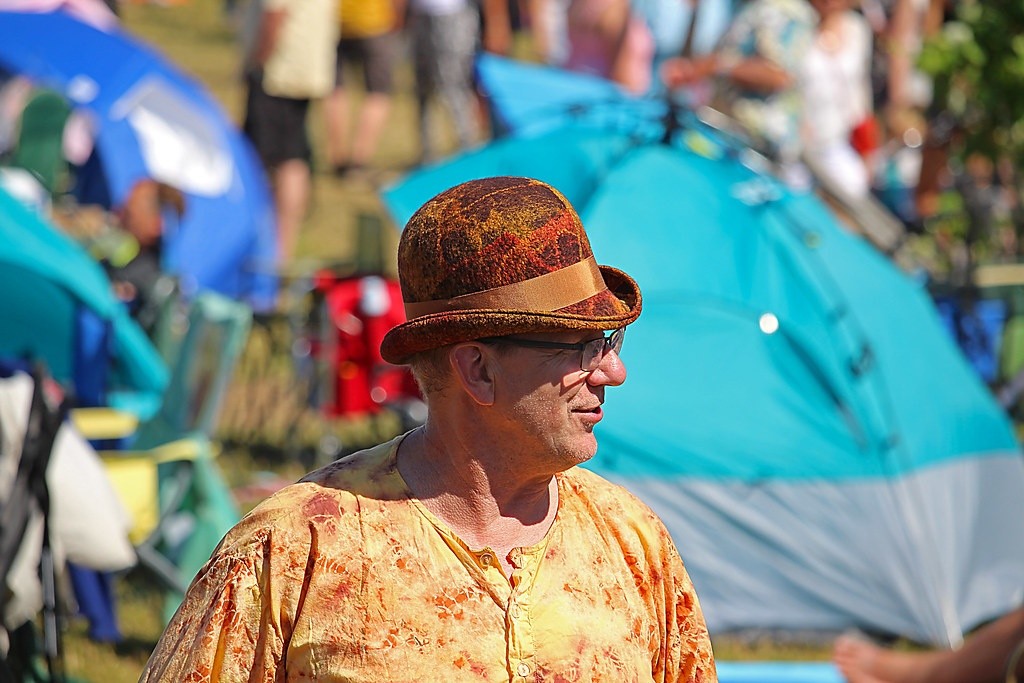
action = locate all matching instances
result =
[386,85,1022,647]
[0,5,281,325]
[0,178,240,614]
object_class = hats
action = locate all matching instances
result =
[379,176,642,368]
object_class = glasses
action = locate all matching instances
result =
[476,323,626,371]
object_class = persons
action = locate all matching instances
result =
[832,606,1024,683]
[132,178,718,679]
[204,0,917,284]
